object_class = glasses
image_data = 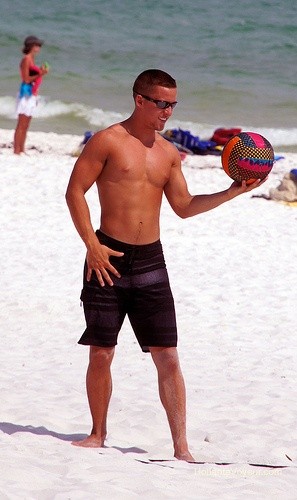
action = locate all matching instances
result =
[139,93,178,109]
[36,44,41,47]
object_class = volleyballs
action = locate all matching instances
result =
[218,133,275,187]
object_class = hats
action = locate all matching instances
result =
[25,36,44,44]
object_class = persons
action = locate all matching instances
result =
[13,36,48,154]
[65,68,268,461]
